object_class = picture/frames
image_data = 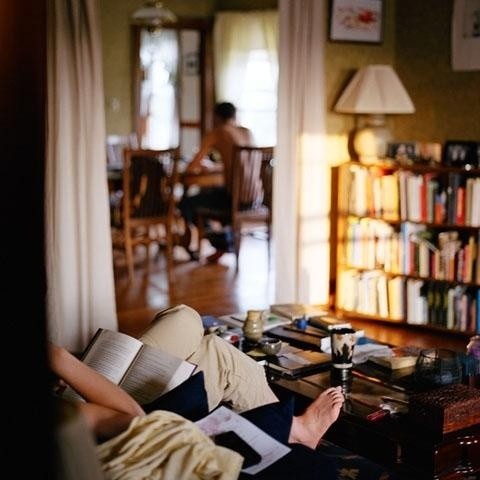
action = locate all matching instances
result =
[330,0,385,44]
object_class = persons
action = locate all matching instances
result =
[178,101,264,263]
[46,304,345,452]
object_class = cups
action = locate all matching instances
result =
[331,328,355,382]
[257,338,283,356]
[296,319,306,329]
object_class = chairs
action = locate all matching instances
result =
[106,132,275,280]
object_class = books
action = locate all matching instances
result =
[267,347,334,379]
[219,306,292,332]
[339,166,480,334]
[199,311,226,331]
[53,327,198,419]
[272,302,326,319]
[310,312,352,331]
[369,343,436,370]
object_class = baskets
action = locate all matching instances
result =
[415,349,462,391]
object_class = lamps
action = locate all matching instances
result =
[133,0,178,37]
[333,64,416,165]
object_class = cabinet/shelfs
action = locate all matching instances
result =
[328,165,480,333]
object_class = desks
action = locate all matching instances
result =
[215,312,479,480]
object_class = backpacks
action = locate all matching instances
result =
[120,156,171,217]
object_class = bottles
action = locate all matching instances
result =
[242,310,264,342]
